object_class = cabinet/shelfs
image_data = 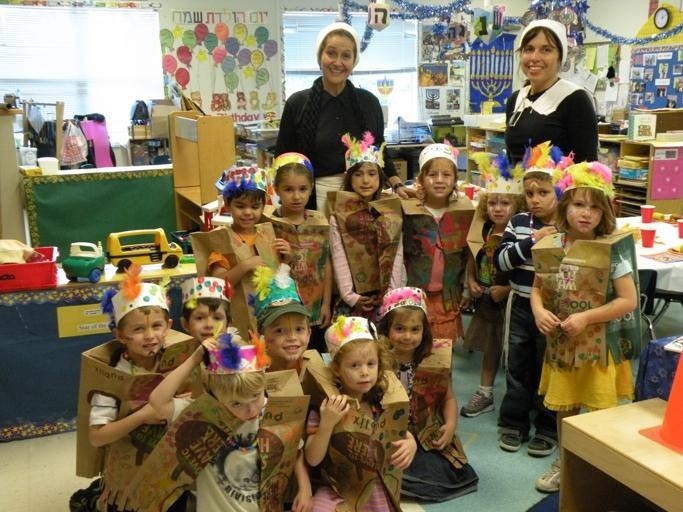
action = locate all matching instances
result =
[127,137,171,167]
[467,122,682,218]
[0,107,31,246]
[167,111,236,233]
[234,134,273,170]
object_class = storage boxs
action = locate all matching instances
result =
[148,97,178,138]
[392,157,408,180]
[428,125,467,147]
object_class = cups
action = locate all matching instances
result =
[464,185,474,200]
[18,147,38,167]
[640,204,656,223]
[640,227,657,248]
[37,157,59,176]
[677,219,683,239]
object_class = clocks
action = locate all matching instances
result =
[653,6,670,28]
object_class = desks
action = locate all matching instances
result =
[0,256,206,442]
[555,393,682,511]
[17,164,177,246]
[614,200,681,349]
[382,139,432,151]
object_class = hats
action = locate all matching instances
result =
[257,300,312,334]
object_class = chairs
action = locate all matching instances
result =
[638,266,662,343]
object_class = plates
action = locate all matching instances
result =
[248,128,278,137]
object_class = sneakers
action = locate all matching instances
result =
[460,390,494,416]
[528,436,557,456]
[536,465,560,492]
[500,428,522,451]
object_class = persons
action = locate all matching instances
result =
[497,141,574,458]
[70,261,478,509]
[260,150,332,353]
[190,164,294,343]
[530,161,653,492]
[504,19,600,164]
[399,140,471,344]
[461,152,526,417]
[274,22,423,211]
[324,131,403,332]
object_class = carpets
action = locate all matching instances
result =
[407,283,681,512]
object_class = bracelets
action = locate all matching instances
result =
[394,183,405,192]
